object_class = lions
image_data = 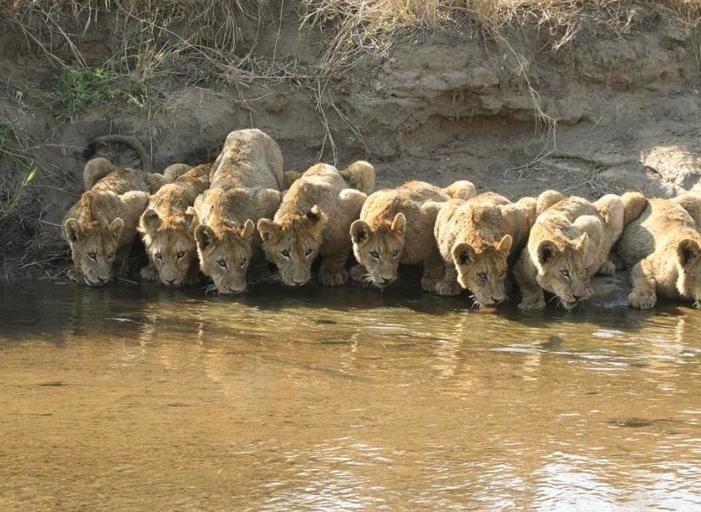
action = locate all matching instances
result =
[62,129,701,310]
